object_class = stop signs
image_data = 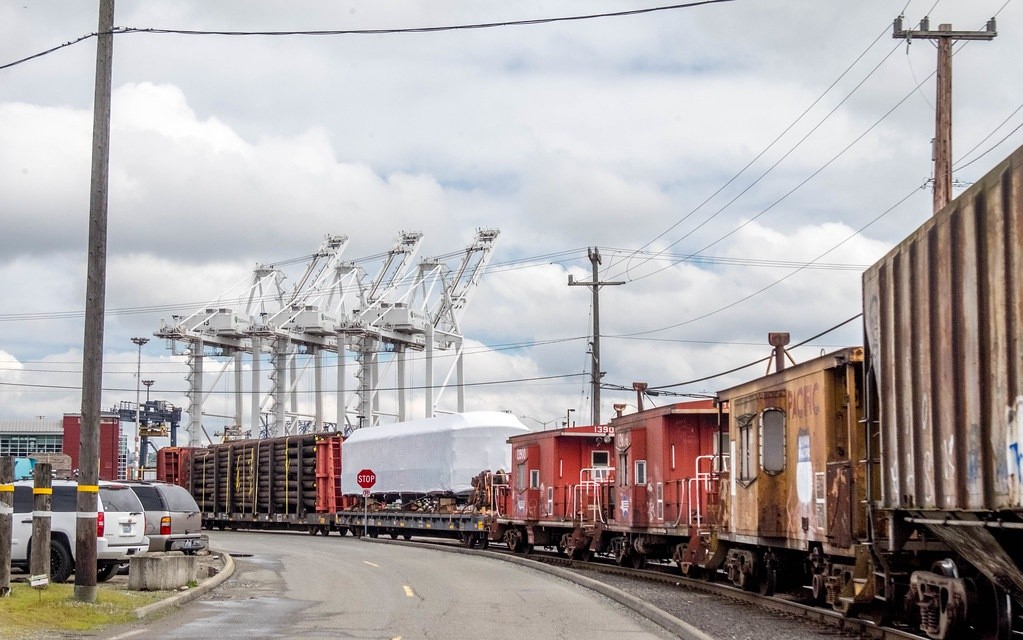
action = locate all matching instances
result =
[356,468,378,490]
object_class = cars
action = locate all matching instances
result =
[110,478,210,577]
[12,480,150,586]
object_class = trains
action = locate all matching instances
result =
[163,147,1023,640]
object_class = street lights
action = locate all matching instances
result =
[142,380,155,471]
[131,337,151,481]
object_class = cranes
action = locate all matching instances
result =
[148,225,504,452]
